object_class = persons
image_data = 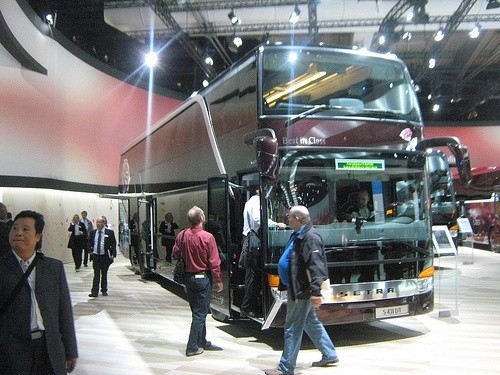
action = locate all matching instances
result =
[0,202,14,256]
[129,212,179,264]
[264,206,339,375]
[326,214,409,284]
[205,212,225,246]
[172,206,224,357]
[0,210,79,375]
[88,217,116,297]
[80,211,110,271]
[67,214,88,272]
[239,185,286,317]
[346,188,378,222]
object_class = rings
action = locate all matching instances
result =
[314,303,316,304]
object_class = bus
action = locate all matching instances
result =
[393,147,459,250]
[452,165,500,254]
[98,41,472,329]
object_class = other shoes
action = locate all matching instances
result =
[265,366,294,375]
[186,348,204,356]
[102,292,107,296]
[88,293,98,297]
[198,341,211,348]
[312,355,339,366]
[240,309,263,318]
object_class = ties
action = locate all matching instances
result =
[97,231,102,255]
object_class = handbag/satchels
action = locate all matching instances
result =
[239,229,261,268]
[173,259,185,284]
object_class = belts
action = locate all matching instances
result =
[31,331,45,340]
